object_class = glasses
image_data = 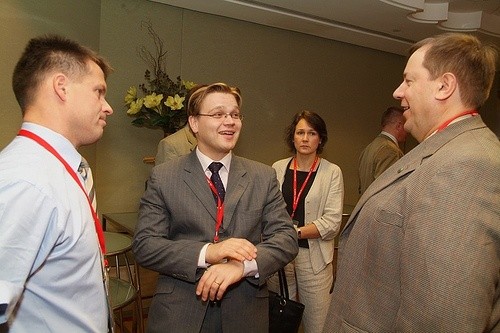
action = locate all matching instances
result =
[195,113,244,122]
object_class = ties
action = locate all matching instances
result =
[209,162,225,206]
[78,156,115,333]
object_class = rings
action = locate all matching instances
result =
[214,281,220,286]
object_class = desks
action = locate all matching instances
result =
[102,211,263,333]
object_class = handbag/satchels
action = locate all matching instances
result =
[266,265,305,333]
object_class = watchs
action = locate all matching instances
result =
[296,227,302,239]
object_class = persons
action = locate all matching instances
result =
[358,106,410,204]
[321,31,500,333]
[154,125,198,165]
[0,34,116,333]
[272,110,344,333]
[130,83,299,333]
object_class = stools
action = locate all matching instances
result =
[103,230,144,333]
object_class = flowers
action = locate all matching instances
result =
[124,16,198,139]
